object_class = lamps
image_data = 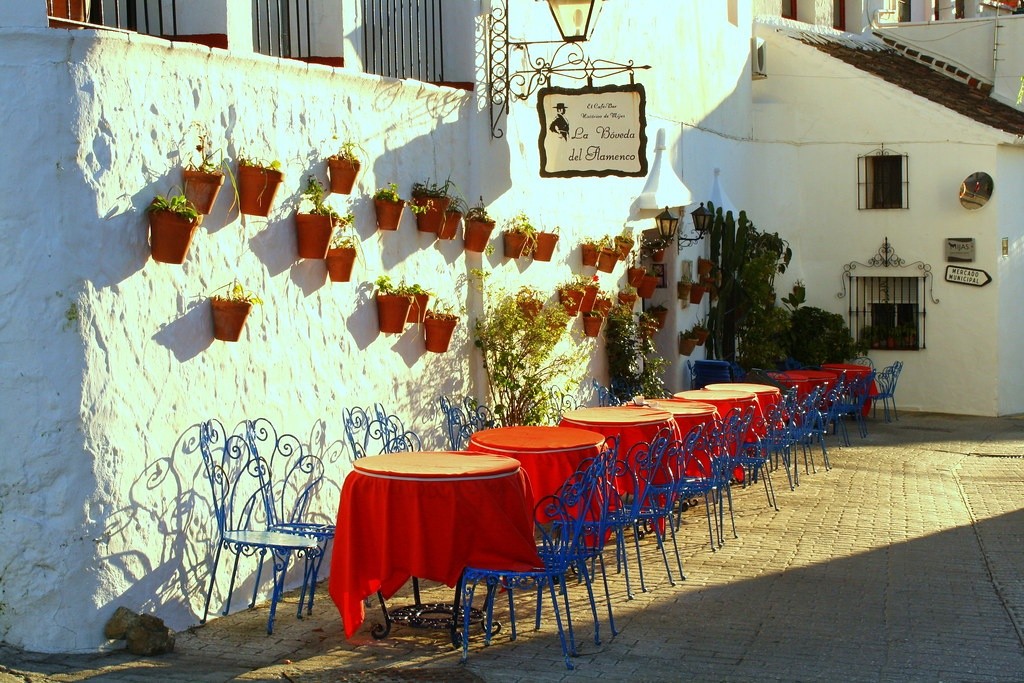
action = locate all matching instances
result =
[677,201,715,255]
[486,0,610,46]
[655,204,679,242]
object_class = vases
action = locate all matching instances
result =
[579,286,599,312]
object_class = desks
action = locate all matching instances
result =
[558,406,684,541]
[328,450,545,643]
[821,363,879,417]
[768,370,838,428]
[671,389,767,487]
[466,426,621,585]
[622,398,726,508]
[704,383,789,439]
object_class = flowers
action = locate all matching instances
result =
[571,271,601,288]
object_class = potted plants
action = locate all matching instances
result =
[437,195,471,242]
[464,196,496,253]
[326,225,369,282]
[675,256,717,357]
[374,274,461,353]
[210,276,265,343]
[412,173,466,233]
[502,208,670,338]
[144,184,203,265]
[178,120,248,231]
[295,174,355,260]
[236,144,287,218]
[326,134,372,197]
[372,181,437,231]
[856,322,917,348]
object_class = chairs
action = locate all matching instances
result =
[194,356,904,677]
